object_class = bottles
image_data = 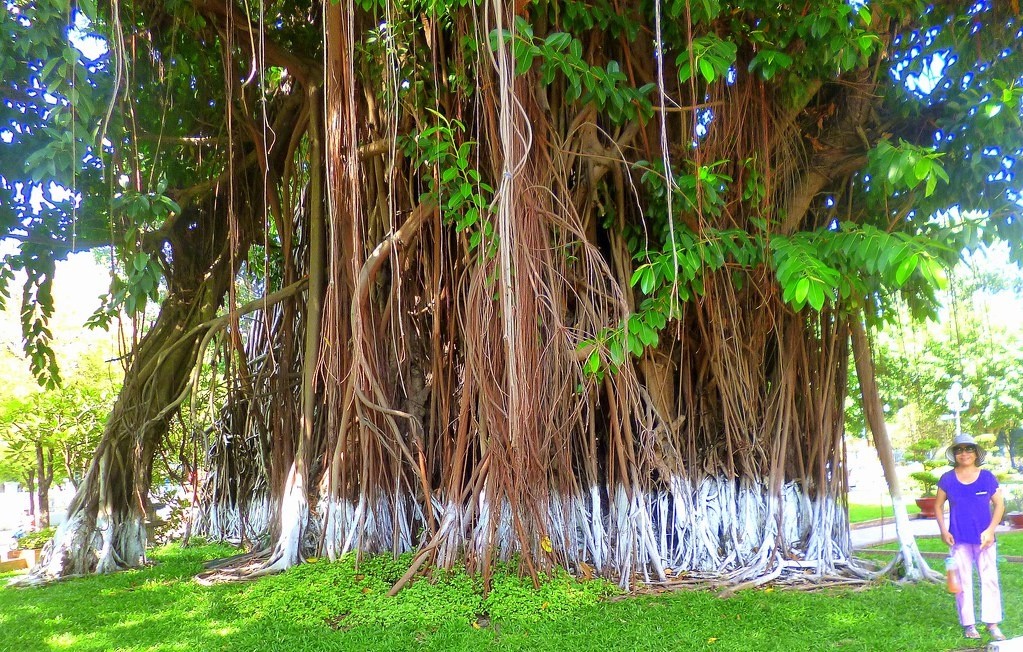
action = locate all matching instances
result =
[946,558,962,593]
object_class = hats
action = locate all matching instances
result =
[945,433,987,466]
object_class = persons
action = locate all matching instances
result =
[21,509,35,536]
[933,434,1006,640]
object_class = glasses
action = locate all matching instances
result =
[953,445,976,454]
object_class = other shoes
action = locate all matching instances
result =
[964,625,981,639]
[985,626,1006,641]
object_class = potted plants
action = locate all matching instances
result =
[18,528,56,570]
[1006,488,1023,528]
[907,439,949,517]
